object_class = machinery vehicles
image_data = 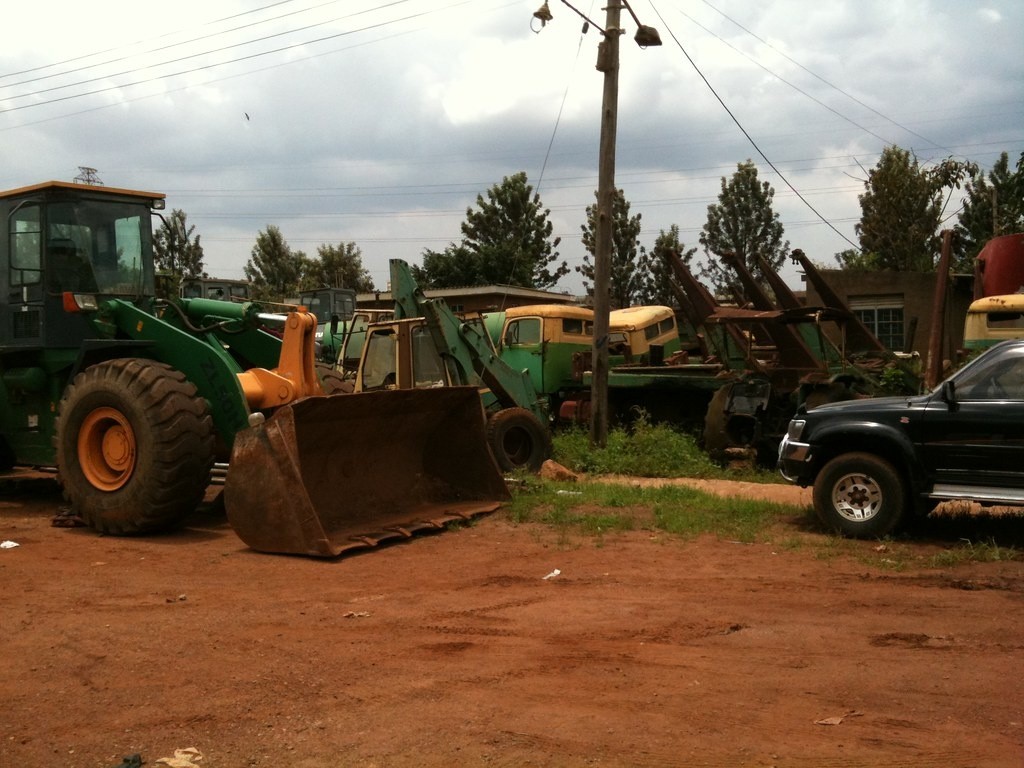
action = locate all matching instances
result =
[0,180,514,558]
[310,234,1024,476]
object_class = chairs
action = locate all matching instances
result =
[46,237,100,294]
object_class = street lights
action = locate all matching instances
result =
[532,0,664,451]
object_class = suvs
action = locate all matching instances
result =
[779,340,1023,537]
